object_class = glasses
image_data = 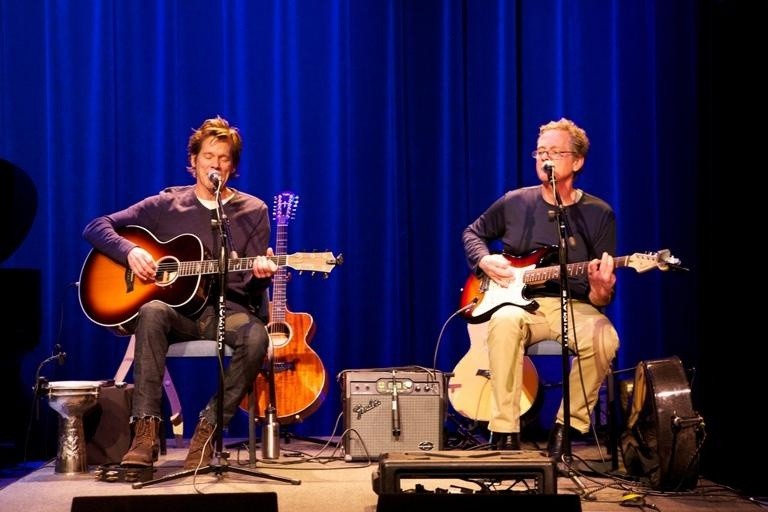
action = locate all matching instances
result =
[532,150,577,160]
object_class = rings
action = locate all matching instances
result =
[259,270,263,274]
[590,268,598,274]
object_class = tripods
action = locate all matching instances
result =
[224,271,342,451]
[133,185,301,489]
[545,167,622,498]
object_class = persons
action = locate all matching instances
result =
[459,118,623,475]
[80,114,279,469]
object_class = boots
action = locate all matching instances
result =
[183,418,217,469]
[500,433,519,451]
[546,424,577,476]
[120,417,160,466]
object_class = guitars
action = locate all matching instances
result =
[77,224,343,339]
[618,356,699,491]
[459,245,680,324]
[446,321,544,426]
[237,192,329,426]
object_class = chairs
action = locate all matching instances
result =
[113,331,234,447]
[524,340,570,356]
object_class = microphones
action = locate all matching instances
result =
[208,170,223,186]
[391,384,401,437]
[541,161,555,171]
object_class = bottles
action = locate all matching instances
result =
[261,406,280,459]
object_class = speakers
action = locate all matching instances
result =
[343,372,449,462]
